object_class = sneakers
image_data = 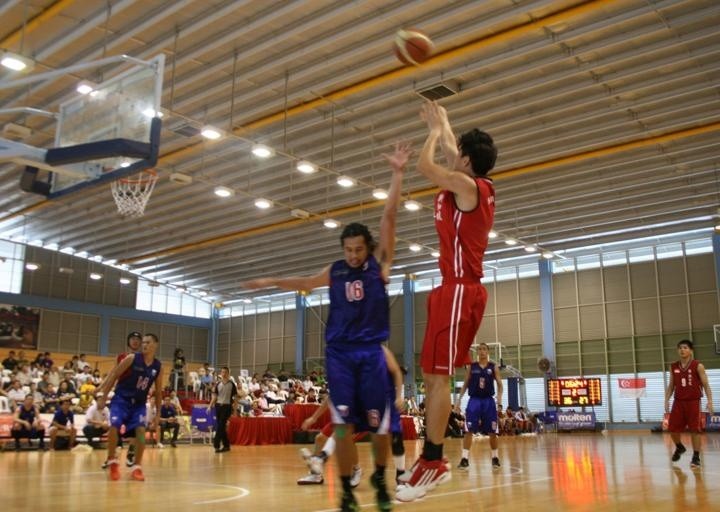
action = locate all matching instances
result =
[215,447,230,452]
[297,474,324,484]
[395,455,451,502]
[492,457,500,468]
[691,454,701,467]
[342,495,359,511]
[370,471,393,511]
[156,441,178,448]
[101,451,146,481]
[300,447,324,475]
[672,445,686,461]
[350,467,362,487]
[457,458,469,470]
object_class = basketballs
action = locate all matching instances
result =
[394,27,434,67]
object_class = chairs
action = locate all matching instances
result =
[0,402,219,446]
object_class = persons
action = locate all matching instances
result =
[235,135,414,512]
[1,350,126,452]
[393,98,497,503]
[664,339,716,470]
[300,332,407,495]
[102,329,144,471]
[146,349,330,453]
[453,342,505,473]
[295,393,365,489]
[400,396,539,439]
[96,333,165,485]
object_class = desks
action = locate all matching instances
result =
[352,415,418,442]
[283,404,333,436]
[225,416,294,446]
